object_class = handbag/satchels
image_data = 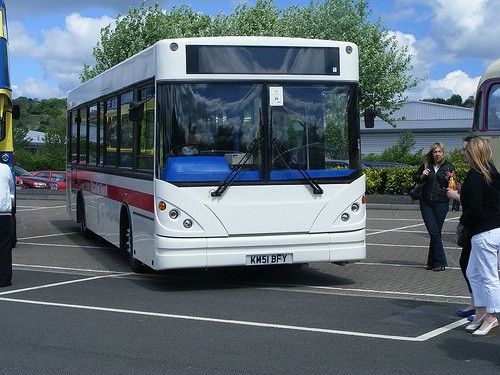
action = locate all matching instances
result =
[454,222,469,247]
[410,163,426,200]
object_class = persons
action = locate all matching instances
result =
[0,162,12,288]
[447,135,480,321]
[456,137,500,337]
[413,143,455,271]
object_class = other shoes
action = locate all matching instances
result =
[456,309,475,317]
[468,314,476,321]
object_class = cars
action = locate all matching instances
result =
[34,170,66,189]
[14,165,58,190]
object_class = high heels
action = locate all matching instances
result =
[425,265,433,270]
[465,314,487,331]
[432,265,445,271]
[472,318,500,336]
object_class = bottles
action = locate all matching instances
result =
[448,173,456,190]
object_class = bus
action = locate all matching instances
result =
[470,57,500,173]
[0,0,21,249]
[65,35,375,274]
